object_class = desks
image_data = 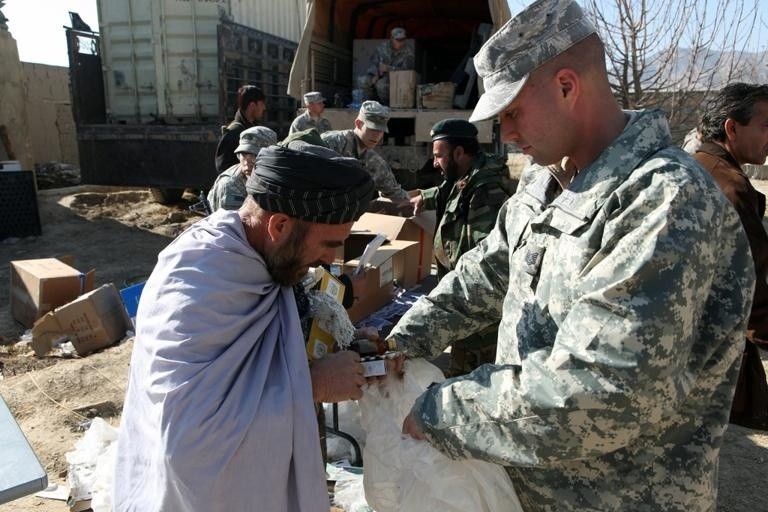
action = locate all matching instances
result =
[324,273,442,467]
[1,394,49,504]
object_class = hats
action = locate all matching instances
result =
[430,118,478,142]
[358,100,391,133]
[390,27,407,38]
[304,92,327,105]
[233,125,277,154]
[467,1,597,123]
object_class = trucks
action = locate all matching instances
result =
[59,0,299,186]
[290,3,516,186]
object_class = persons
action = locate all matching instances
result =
[320,98,409,206]
[396,116,520,378]
[691,81,768,431]
[287,89,333,137]
[215,85,267,174]
[360,1,758,510]
[107,136,387,512]
[358,27,416,107]
[204,123,277,213]
[680,127,704,156]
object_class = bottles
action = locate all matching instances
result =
[349,337,397,354]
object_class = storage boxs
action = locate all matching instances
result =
[388,69,417,109]
[9,255,150,356]
[417,81,455,110]
[293,201,438,364]
